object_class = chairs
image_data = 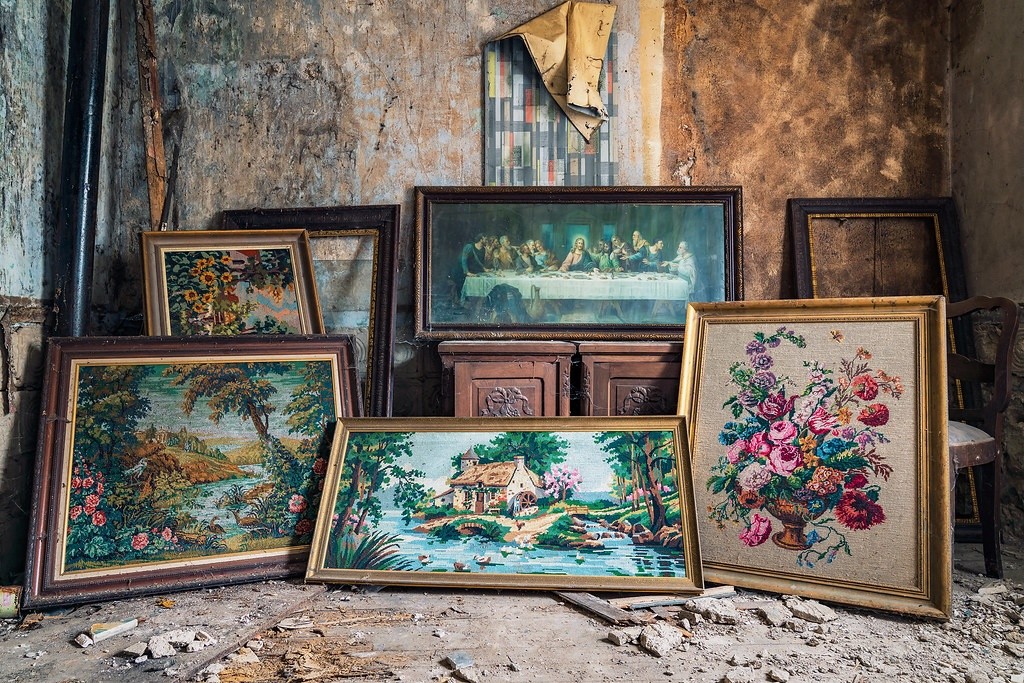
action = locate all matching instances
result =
[946,296,1021,577]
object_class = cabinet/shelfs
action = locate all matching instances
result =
[580,341,684,415]
[439,340,576,417]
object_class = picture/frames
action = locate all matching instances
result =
[139,228,326,336]
[22,333,364,615]
[676,296,952,620]
[414,185,744,340]
[304,415,706,595]
[790,195,985,544]
[218,204,400,418]
